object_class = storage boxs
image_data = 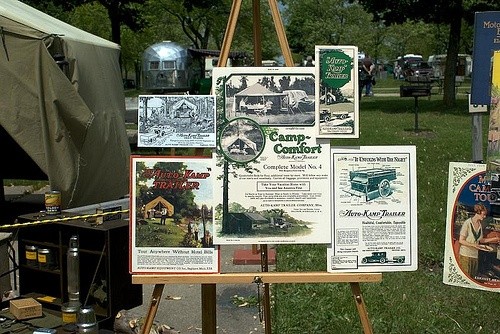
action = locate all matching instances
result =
[10,297,42,320]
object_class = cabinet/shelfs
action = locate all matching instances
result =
[17,211,143,319]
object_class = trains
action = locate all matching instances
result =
[140,41,204,95]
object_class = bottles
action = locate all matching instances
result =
[62,301,82,332]
[76,306,99,334]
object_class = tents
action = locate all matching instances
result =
[0,0,130,210]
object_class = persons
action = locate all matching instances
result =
[359,55,375,97]
[265,98,273,115]
[393,64,402,79]
[239,98,249,116]
[306,56,315,67]
[459,203,498,275]
[140,204,167,225]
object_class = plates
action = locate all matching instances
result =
[479,245,493,252]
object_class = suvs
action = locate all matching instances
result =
[403,59,434,80]
